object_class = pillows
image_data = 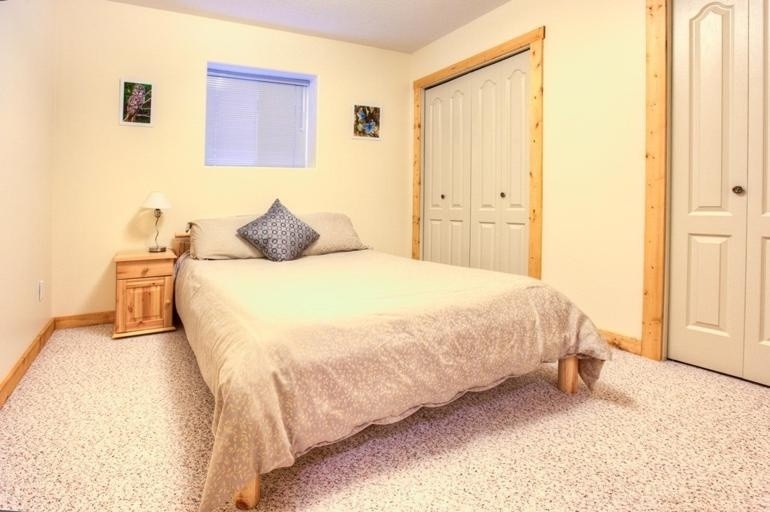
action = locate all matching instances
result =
[186,198,368,260]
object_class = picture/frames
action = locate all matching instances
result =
[118,78,155,127]
[350,103,382,141]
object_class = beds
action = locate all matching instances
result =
[174,231,582,512]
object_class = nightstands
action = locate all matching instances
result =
[111,249,178,341]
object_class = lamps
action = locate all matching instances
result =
[141,193,171,254]
